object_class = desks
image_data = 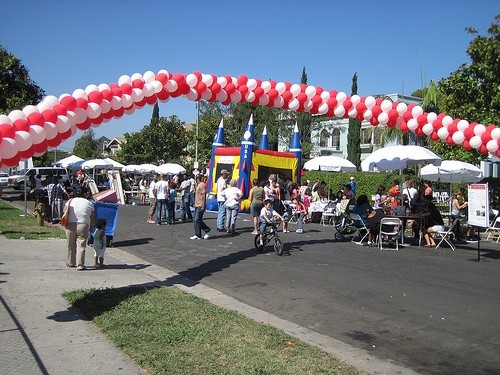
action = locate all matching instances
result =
[125,191,148,204]
[386,214,426,248]
[307,200,332,222]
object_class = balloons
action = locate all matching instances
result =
[162,72,306,112]
[304,85,500,158]
[0,68,167,170]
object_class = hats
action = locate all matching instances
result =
[221,169,230,174]
[350,177,355,179]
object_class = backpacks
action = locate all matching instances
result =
[304,197,310,207]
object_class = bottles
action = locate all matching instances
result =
[406,208,409,216]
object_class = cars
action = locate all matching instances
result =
[0,172,11,186]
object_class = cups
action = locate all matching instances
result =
[386,211,390,215]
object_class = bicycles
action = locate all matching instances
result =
[255,217,284,257]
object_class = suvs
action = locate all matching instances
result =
[6,167,69,190]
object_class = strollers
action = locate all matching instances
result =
[332,211,358,240]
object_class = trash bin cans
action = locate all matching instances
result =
[88,198,120,245]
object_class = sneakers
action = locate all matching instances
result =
[204,229,212,239]
[190,235,201,239]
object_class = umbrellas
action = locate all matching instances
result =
[358,145,442,206]
[303,156,357,201]
[54,154,187,175]
[418,160,483,214]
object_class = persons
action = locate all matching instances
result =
[216,169,244,234]
[258,199,284,247]
[189,174,212,239]
[62,185,95,270]
[248,175,327,235]
[139,172,196,226]
[335,175,477,248]
[93,219,106,269]
[29,172,88,221]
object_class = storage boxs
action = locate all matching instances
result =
[90,189,119,203]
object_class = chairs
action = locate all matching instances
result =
[320,208,337,227]
[289,204,300,225]
[349,212,369,246]
[375,217,401,251]
[281,199,292,210]
[434,217,460,251]
[483,208,499,233]
[486,217,500,242]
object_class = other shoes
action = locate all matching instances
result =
[260,244,263,248]
[367,242,371,244]
[94,263,99,266]
[217,227,236,234]
[251,231,258,234]
[466,236,478,242]
[283,230,290,232]
[147,217,193,226]
[99,264,105,267]
[66,262,75,267]
[296,229,303,233]
[423,244,436,248]
[270,231,274,234]
[472,234,482,240]
[76,265,87,270]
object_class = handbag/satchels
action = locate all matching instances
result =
[411,198,416,206]
[142,188,147,193]
[60,198,74,226]
[224,197,238,209]
[283,210,290,222]
